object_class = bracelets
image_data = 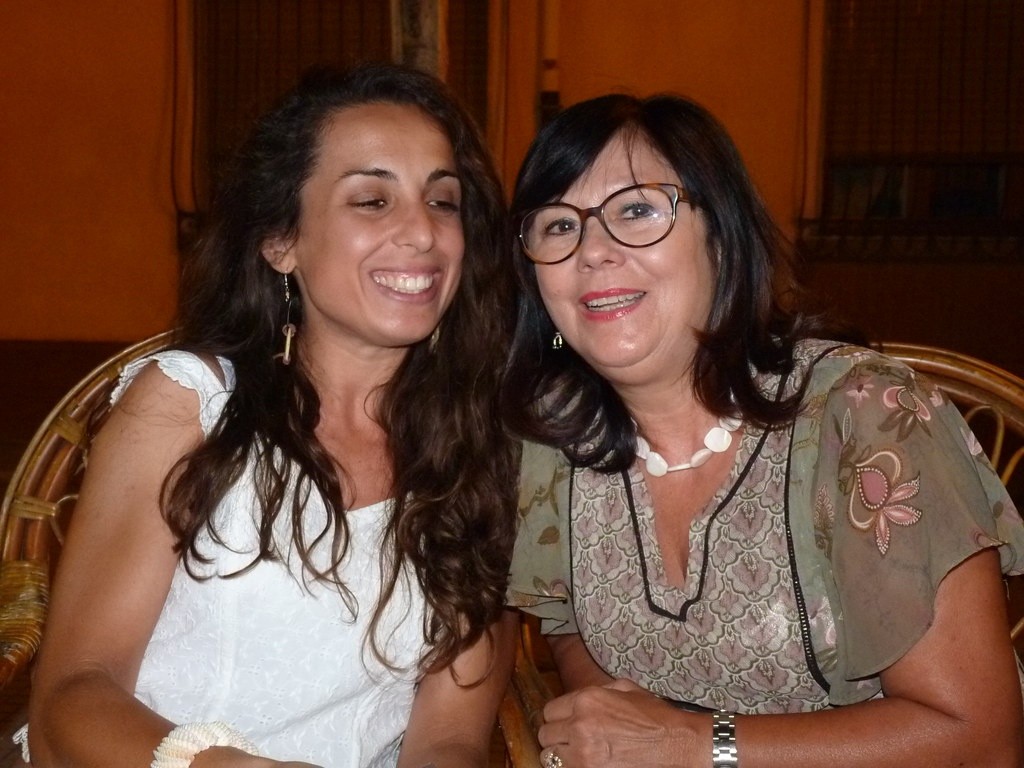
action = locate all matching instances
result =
[150,720,259,768]
[711,710,738,768]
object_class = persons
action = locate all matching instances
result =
[27,47,522,768]
[497,91,1024,768]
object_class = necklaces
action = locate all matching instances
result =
[621,357,756,476]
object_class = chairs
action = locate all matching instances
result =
[0,326,1024,768]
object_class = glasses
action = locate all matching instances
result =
[518,183,711,265]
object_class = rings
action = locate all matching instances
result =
[544,748,563,768]
